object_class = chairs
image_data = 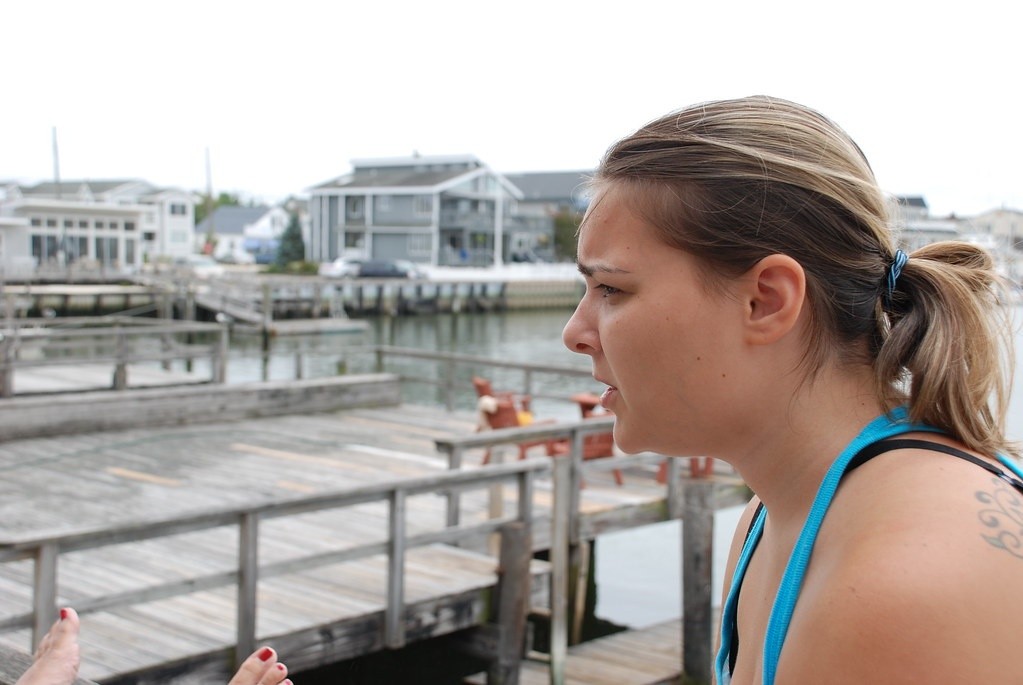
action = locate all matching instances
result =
[473,376,623,488]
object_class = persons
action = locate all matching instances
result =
[12,604,294,685]
[565,94,1023,685]
